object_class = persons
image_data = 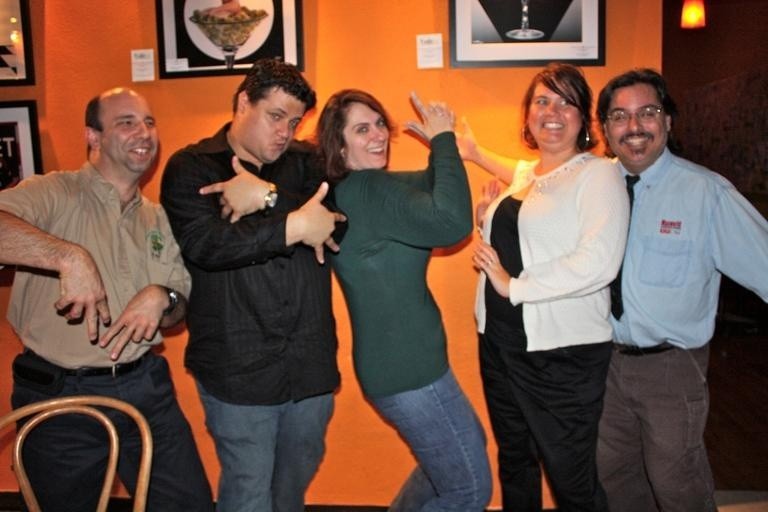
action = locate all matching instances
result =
[0,87,214,512]
[453,68,766,512]
[467,62,631,511]
[159,59,348,512]
[314,88,493,512]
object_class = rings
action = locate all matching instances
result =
[487,261,492,264]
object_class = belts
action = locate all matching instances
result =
[613,342,674,356]
[65,350,151,378]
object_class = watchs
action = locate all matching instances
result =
[265,184,277,210]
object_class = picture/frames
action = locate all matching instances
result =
[156,0,304,79]
[0,0,36,86]
[449,0,606,67]
[0,99,43,191]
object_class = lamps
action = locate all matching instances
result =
[680,0,706,29]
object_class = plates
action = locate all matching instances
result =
[181,0,277,64]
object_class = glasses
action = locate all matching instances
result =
[607,106,662,121]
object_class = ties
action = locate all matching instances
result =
[611,175,639,320]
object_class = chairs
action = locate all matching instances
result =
[0,395,153,512]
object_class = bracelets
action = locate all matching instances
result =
[163,289,179,316]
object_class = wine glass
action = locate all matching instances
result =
[188,5,270,70]
[505,0,545,41]
